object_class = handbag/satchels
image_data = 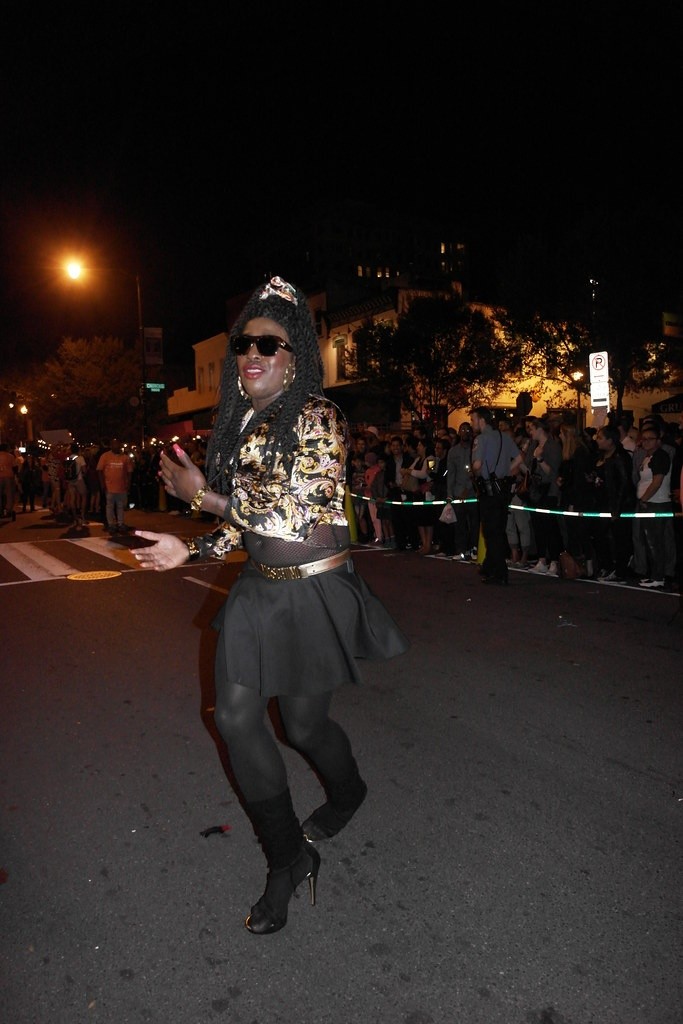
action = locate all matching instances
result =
[439,503,457,524]
[399,473,418,492]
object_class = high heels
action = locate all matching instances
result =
[245,845,321,935]
[301,780,368,843]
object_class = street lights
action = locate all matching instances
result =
[57,252,148,445]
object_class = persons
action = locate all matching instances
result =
[131,278,411,935]
[0,438,206,534]
[347,407,683,589]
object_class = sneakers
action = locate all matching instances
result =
[599,569,627,584]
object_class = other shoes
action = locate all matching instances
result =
[369,536,383,547]
[505,559,525,568]
[546,562,558,575]
[381,537,396,549]
[638,578,665,588]
[528,558,549,573]
[414,547,432,555]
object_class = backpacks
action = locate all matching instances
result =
[62,455,80,480]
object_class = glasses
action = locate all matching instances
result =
[230,333,293,356]
[642,437,656,441]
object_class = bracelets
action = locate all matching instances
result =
[538,458,544,463]
[191,486,212,513]
[182,535,199,561]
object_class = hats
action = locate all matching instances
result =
[364,427,378,438]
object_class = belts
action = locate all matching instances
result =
[250,548,351,580]
